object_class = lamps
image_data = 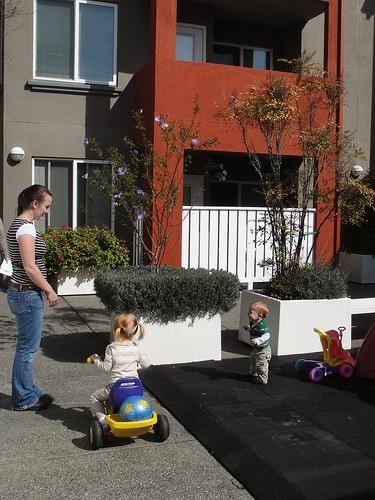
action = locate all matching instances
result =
[352,165,364,177]
[10,147,26,162]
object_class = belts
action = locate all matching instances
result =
[9,281,43,291]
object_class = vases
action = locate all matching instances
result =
[50,267,102,295]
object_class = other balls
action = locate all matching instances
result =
[118,395,153,422]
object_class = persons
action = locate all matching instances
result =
[89,313,152,432]
[242,301,272,384]
[5,184,59,411]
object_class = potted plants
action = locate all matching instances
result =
[213,49,375,356]
[93,264,242,371]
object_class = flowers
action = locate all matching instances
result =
[43,224,130,274]
[82,107,228,273]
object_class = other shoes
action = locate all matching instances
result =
[41,394,54,402]
[255,381,262,384]
[102,423,111,432]
[239,374,257,380]
[25,401,50,411]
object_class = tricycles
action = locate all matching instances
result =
[87,354,170,451]
[295,325,358,382]
